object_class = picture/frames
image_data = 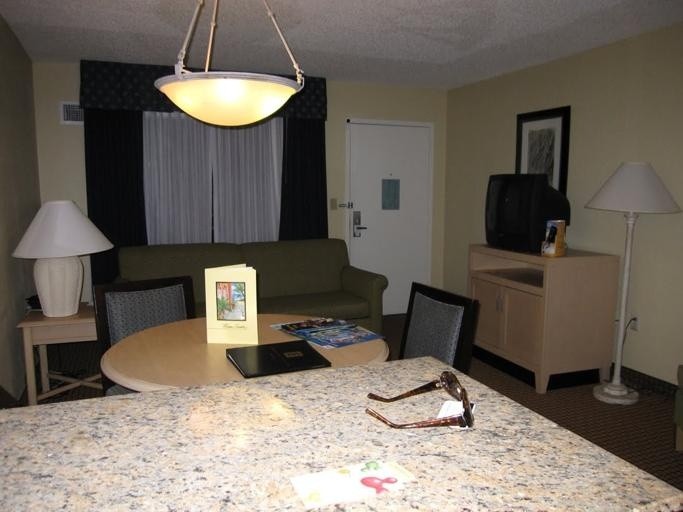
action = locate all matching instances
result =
[514,105,571,196]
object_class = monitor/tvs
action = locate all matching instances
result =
[484,172,572,254]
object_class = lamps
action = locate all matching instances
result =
[12,201,115,316]
[584,160,683,407]
[154,0,305,129]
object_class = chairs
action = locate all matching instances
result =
[399,281,480,373]
[94,273,197,395]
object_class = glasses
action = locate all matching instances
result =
[365,371,474,429]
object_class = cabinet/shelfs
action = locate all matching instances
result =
[468,243,622,395]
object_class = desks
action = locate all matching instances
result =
[101,313,390,392]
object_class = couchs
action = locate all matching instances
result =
[116,238,389,335]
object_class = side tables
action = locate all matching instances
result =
[16,304,104,406]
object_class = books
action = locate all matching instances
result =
[280,317,385,351]
[205,263,259,346]
[226,340,332,379]
